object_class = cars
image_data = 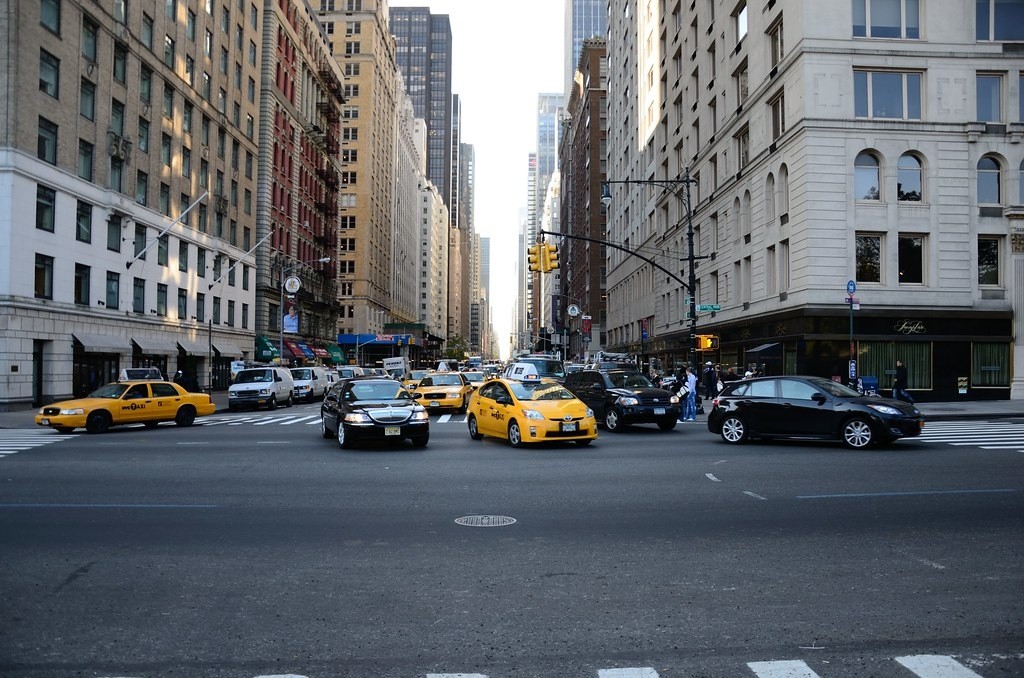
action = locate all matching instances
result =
[466,374,599,447]
[402,369,436,396]
[412,361,475,414]
[707,376,925,450]
[464,356,515,380]
[34,368,216,434]
[325,364,392,395]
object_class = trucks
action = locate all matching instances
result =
[228,367,296,411]
[289,367,330,404]
[320,377,430,449]
[382,356,411,381]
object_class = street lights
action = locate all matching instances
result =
[279,257,331,367]
[600,166,704,412]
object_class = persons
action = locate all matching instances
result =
[649,370,661,388]
[711,365,723,399]
[704,361,716,401]
[683,368,696,421]
[676,373,686,422]
[283,305,298,332]
[892,360,914,405]
[680,361,689,373]
[725,368,738,380]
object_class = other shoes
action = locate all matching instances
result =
[710,397,714,400]
[703,397,708,400]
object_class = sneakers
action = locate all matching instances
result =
[683,417,695,421]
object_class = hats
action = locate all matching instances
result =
[706,361,712,365]
[745,371,753,376]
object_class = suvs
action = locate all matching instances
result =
[563,368,682,432]
[515,357,568,387]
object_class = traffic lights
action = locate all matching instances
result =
[398,339,402,346]
[543,242,560,274]
[694,334,720,353]
[411,338,416,344]
[527,242,542,272]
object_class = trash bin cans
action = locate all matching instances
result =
[858,376,879,396]
